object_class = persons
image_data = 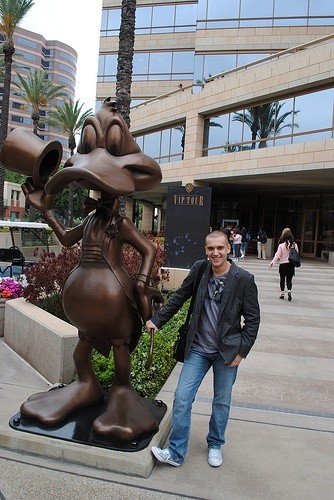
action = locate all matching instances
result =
[220,226,250,259]
[257,228,267,260]
[268,228,299,301]
[144,231,260,467]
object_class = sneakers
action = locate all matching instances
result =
[151,446,181,467]
[208,448,223,466]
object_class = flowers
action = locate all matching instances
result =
[0,278,24,299]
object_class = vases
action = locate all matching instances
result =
[0,297,12,336]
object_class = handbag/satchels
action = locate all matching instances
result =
[172,323,189,363]
[288,242,301,267]
[245,232,251,242]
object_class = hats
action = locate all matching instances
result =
[282,228,291,237]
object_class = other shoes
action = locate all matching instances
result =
[263,257,266,260]
[240,255,244,260]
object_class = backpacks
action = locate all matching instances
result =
[258,231,267,244]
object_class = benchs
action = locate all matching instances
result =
[321,250,334,265]
[0,249,24,267]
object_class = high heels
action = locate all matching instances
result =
[288,292,291,301]
[279,294,284,299]
